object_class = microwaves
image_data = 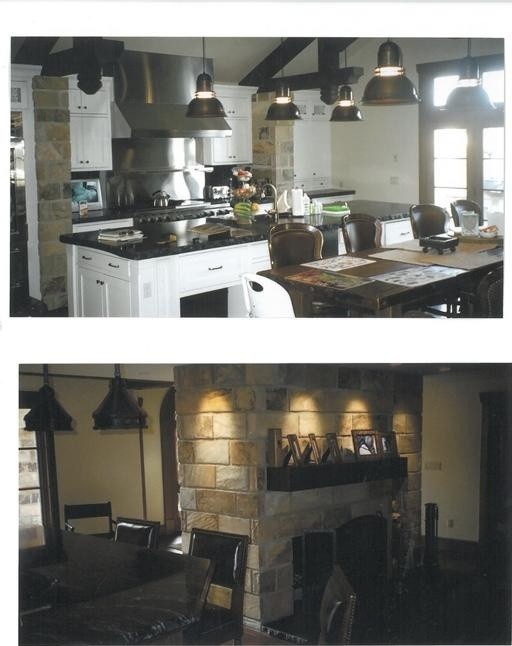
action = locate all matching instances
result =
[71,178,103,213]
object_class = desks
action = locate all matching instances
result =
[256,231,503,317]
[19,524,215,646]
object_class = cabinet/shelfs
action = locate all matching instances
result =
[385,220,412,245]
[77,247,132,317]
[69,89,113,173]
[196,96,253,166]
[184,247,251,294]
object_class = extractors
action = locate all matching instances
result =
[110,51,233,140]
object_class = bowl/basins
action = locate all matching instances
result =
[479,231,498,239]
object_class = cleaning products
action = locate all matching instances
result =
[304,192,310,216]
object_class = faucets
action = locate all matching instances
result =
[260,183,281,224]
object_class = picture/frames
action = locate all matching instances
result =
[379,431,399,458]
[287,434,301,462]
[326,433,341,463]
[309,433,322,464]
[351,429,378,463]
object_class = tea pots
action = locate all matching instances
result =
[153,190,170,208]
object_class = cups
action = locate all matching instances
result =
[462,211,479,236]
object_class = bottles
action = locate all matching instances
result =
[303,193,323,216]
[228,178,234,197]
[205,184,212,200]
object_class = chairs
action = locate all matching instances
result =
[186,527,250,646]
[241,273,296,318]
[115,516,161,550]
[342,214,382,254]
[316,563,358,645]
[450,200,481,227]
[411,204,450,239]
[64,501,117,541]
[268,223,324,269]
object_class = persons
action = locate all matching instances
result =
[358,435,373,455]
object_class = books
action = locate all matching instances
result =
[97,229,145,241]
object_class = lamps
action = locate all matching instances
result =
[185,38,227,119]
[92,364,148,431]
[23,364,72,432]
[330,38,363,122]
[441,38,495,113]
[361,38,421,105]
[264,37,302,121]
